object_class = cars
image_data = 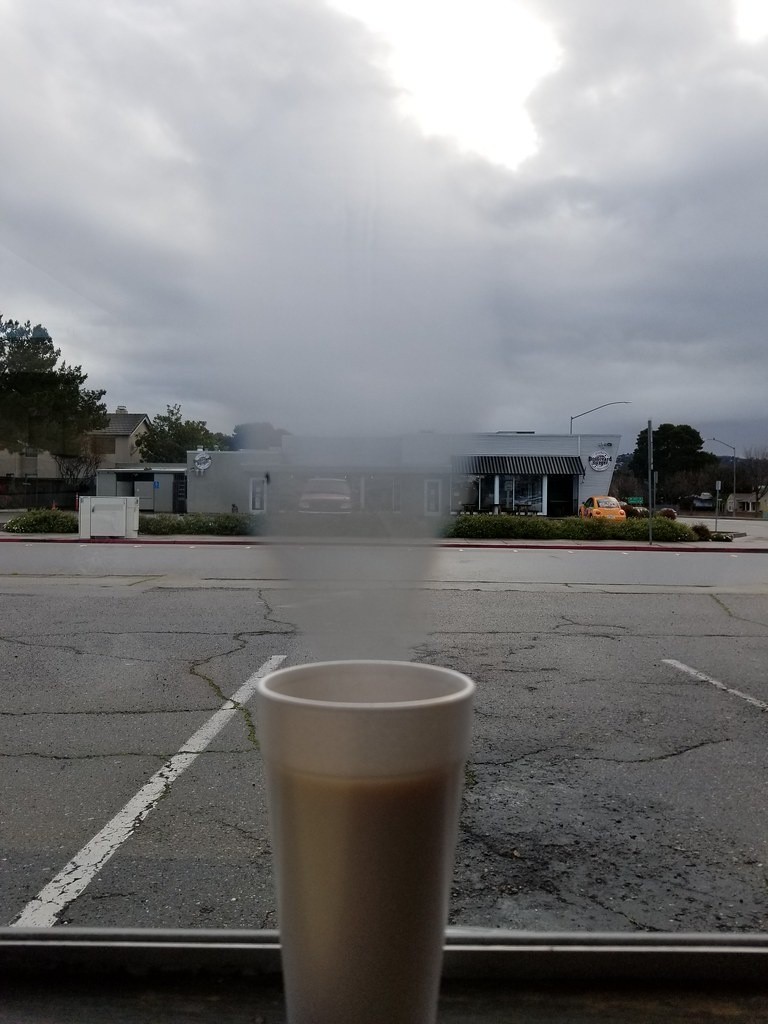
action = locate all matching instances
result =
[579,496,626,524]
[629,503,677,521]
[297,474,354,526]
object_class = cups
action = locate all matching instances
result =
[257,659,475,1023]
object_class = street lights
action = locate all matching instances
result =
[705,438,737,517]
[714,480,721,533]
